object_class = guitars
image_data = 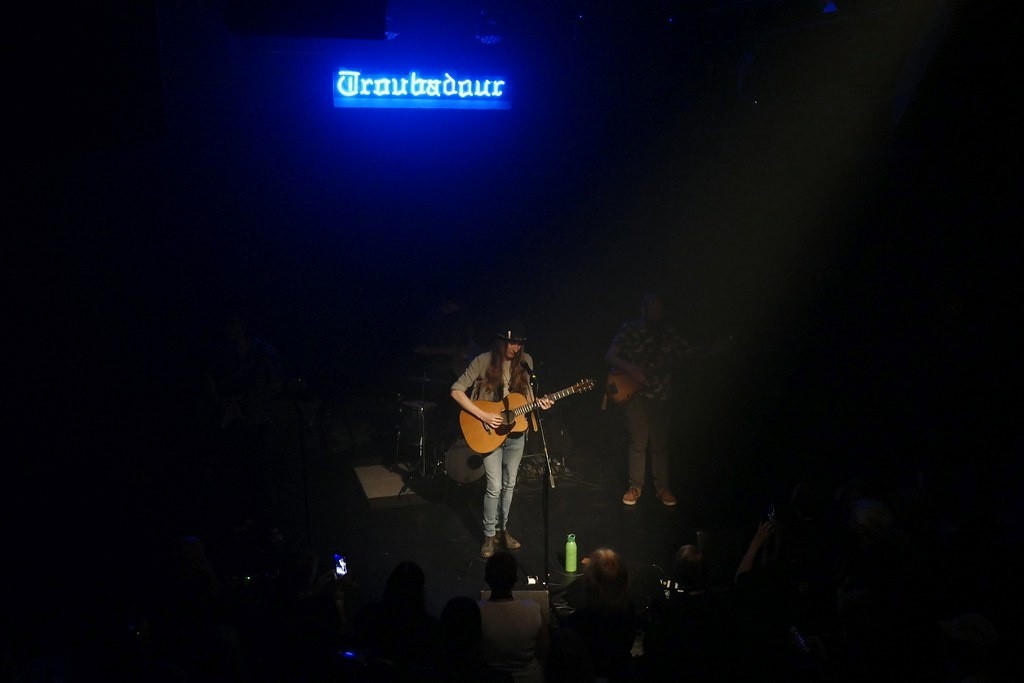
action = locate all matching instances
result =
[605,332,740,406]
[458,377,597,458]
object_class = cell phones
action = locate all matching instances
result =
[333,554,347,578]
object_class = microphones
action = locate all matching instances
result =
[519,360,536,380]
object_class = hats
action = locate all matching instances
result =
[496,322,528,341]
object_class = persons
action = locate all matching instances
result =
[605,297,717,505]
[450,320,557,557]
[1,492,1024,683]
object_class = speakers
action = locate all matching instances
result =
[481,590,550,625]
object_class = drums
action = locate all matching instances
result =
[399,400,440,446]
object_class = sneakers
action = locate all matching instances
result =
[656,489,676,505]
[481,537,495,557]
[496,531,521,549]
[622,487,641,504]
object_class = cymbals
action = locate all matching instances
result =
[405,376,446,385]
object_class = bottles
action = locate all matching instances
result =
[565,534,577,572]
